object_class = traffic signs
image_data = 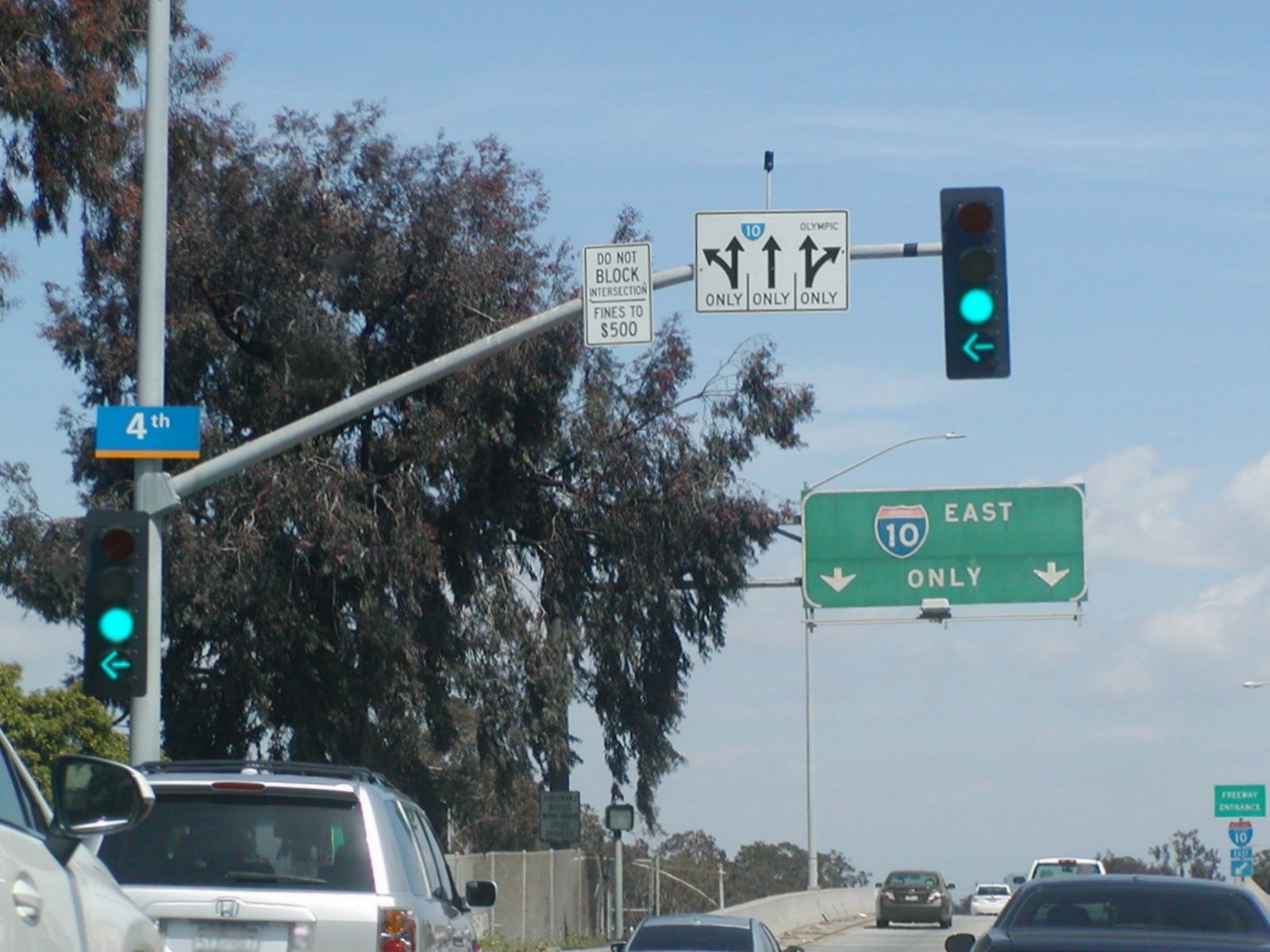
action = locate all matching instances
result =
[799,481,1089,611]
[695,209,852,311]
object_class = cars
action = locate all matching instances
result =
[945,873,1270,952]
[610,915,805,952]
[875,869,956,930]
[0,727,166,952]
[969,883,1013,916]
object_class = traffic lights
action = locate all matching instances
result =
[606,804,634,832]
[940,186,1011,380]
[81,509,150,699]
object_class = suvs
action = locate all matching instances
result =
[81,761,498,952]
[1013,857,1111,923]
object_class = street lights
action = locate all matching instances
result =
[800,430,967,888]
